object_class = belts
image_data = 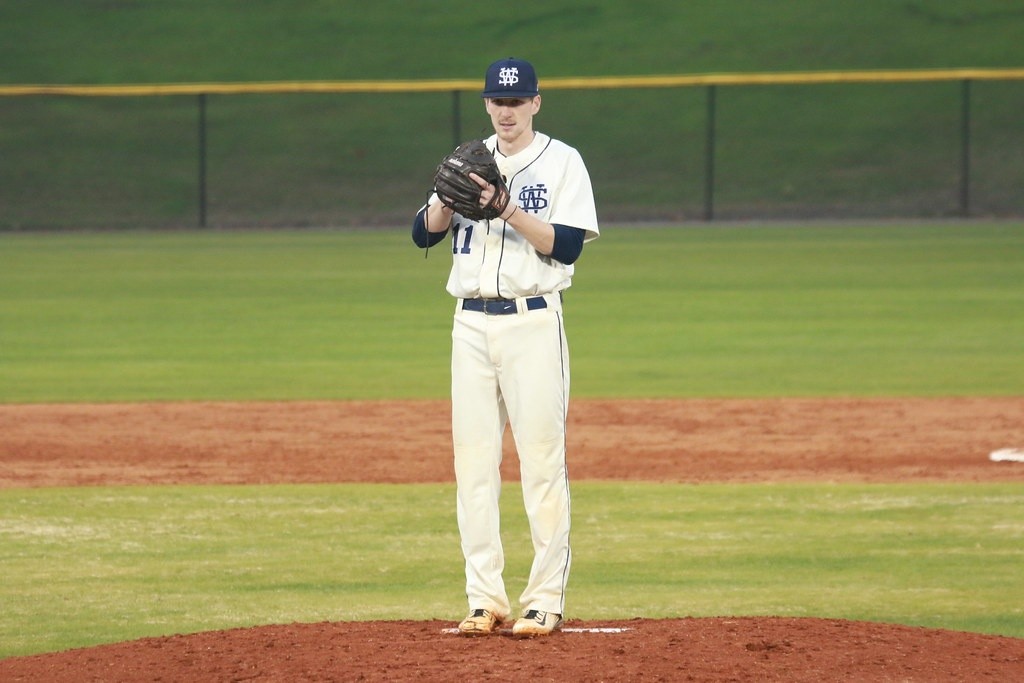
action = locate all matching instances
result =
[462,292,563,315]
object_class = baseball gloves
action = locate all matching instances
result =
[433,138,512,221]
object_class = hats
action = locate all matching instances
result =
[481,56,539,99]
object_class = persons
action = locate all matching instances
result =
[412,59,599,638]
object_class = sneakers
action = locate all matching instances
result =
[458,609,499,634]
[513,609,562,638]
